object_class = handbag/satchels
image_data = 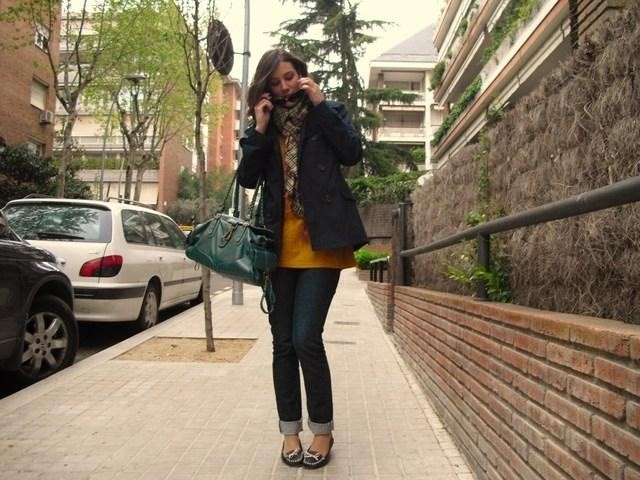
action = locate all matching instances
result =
[185,212,277,286]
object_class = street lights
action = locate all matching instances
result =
[98,73,149,198]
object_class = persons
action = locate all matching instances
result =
[235,48,369,470]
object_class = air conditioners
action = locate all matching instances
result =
[39,109,54,124]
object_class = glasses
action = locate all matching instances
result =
[271,90,305,106]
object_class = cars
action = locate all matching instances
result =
[0,214,78,390]
[3,196,204,330]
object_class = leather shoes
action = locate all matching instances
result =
[303,435,334,468]
[282,438,304,466]
